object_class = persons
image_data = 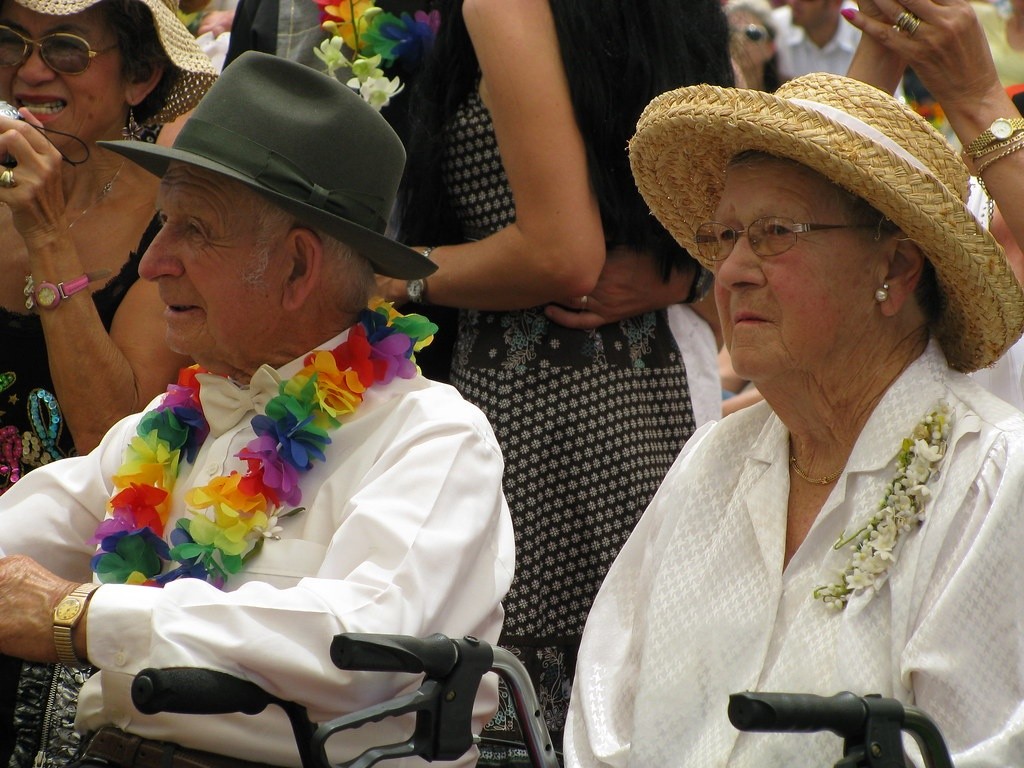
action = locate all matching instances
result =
[380,0,739,768]
[0,53,515,768]
[724,0,1024,312]
[564,69,1024,768]
[0,0,362,498]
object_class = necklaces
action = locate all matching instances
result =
[85,296,438,591]
[788,455,848,485]
[24,159,126,310]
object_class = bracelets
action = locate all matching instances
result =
[964,131,1024,158]
[967,141,1024,222]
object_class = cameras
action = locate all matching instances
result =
[0,99,26,168]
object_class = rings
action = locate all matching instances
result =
[0,167,15,188]
[581,296,588,310]
[894,9,922,37]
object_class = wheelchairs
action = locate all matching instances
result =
[130,665,331,768]
[307,631,958,768]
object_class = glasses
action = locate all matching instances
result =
[694,216,894,261]
[0,25,125,74]
[727,25,770,41]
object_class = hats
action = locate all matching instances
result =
[95,52,440,281]
[628,71,1024,374]
[12,0,220,126]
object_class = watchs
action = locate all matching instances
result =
[35,270,110,312]
[963,118,1024,162]
[405,247,435,306]
[52,582,103,669]
[677,259,714,305]
